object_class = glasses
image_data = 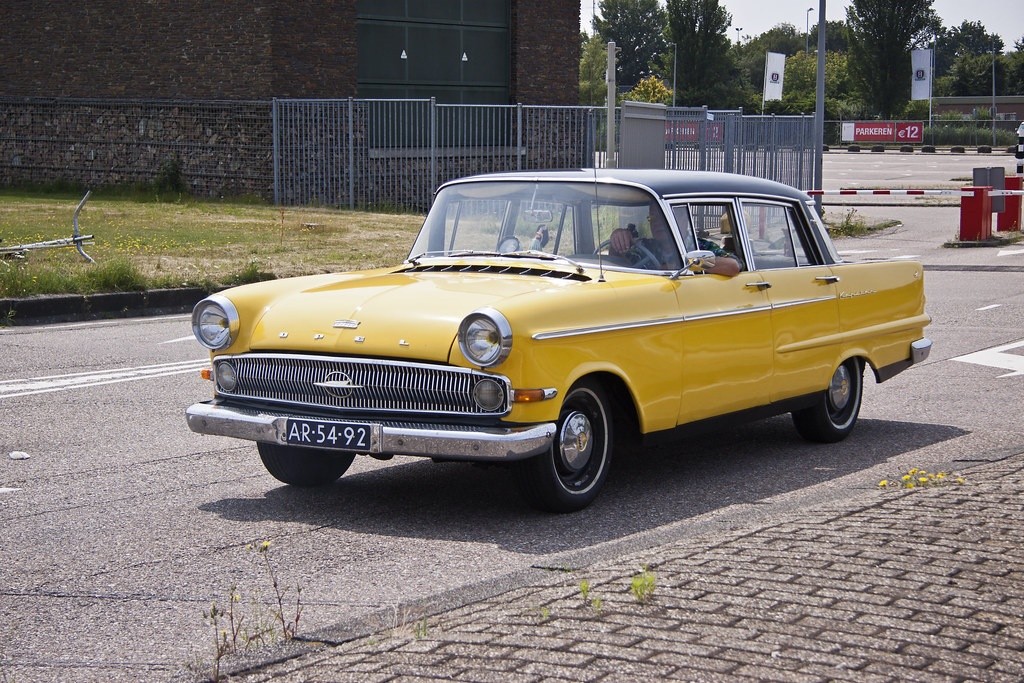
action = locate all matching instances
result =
[646,215,656,222]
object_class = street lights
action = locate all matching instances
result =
[669,42,677,106]
[805,7,814,55]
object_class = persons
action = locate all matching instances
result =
[609,198,745,277]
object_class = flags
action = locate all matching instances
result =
[764,52,786,101]
[911,49,930,100]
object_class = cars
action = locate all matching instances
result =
[182,169,936,512]
